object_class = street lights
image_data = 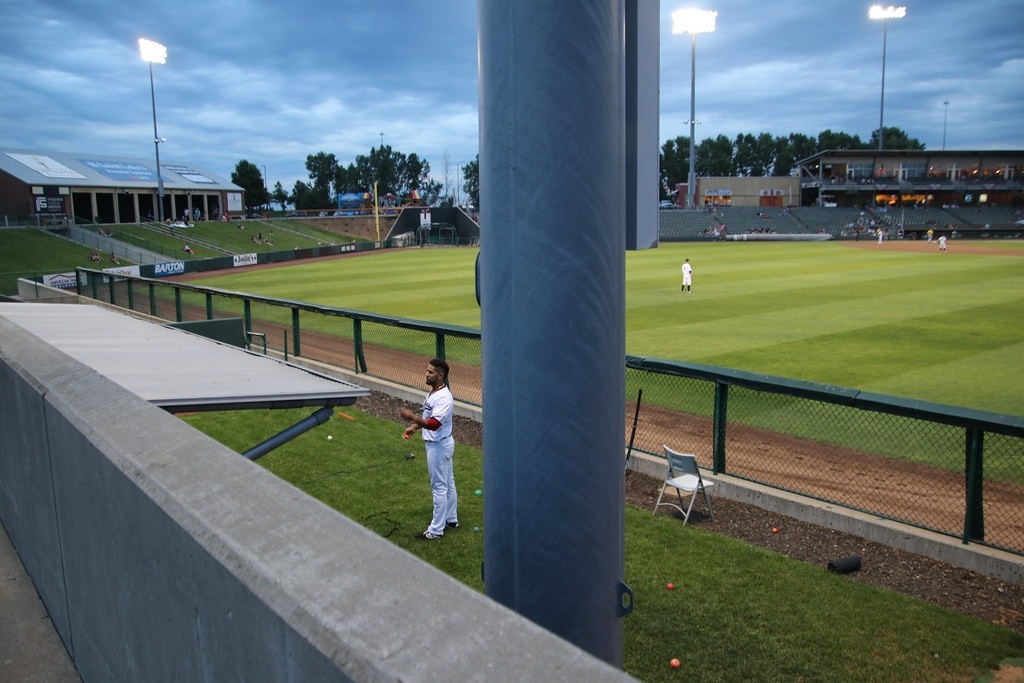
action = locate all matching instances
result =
[870,5,906,150]
[262,164,267,188]
[943,101,950,151]
[379,132,385,145]
[671,8,717,209]
[139,39,167,223]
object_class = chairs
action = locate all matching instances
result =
[652,445,715,528]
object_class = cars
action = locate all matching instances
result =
[660,200,673,211]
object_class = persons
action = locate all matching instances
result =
[938,234,947,252]
[682,259,692,292]
[878,230,883,245]
[194,207,200,222]
[89,249,120,265]
[751,227,776,234]
[842,205,889,243]
[167,215,189,229]
[927,228,934,243]
[705,224,726,236]
[399,358,459,540]
[184,207,189,221]
[251,231,275,246]
[184,242,194,254]
[99,226,116,237]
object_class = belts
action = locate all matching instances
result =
[424,437,447,443]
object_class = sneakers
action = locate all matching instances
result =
[445,519,460,530]
[415,530,445,541]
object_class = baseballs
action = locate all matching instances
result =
[670,658,680,668]
[667,582,674,590]
[404,434,409,439]
[474,489,482,496]
[327,435,333,441]
[474,527,479,531]
[773,528,777,533]
